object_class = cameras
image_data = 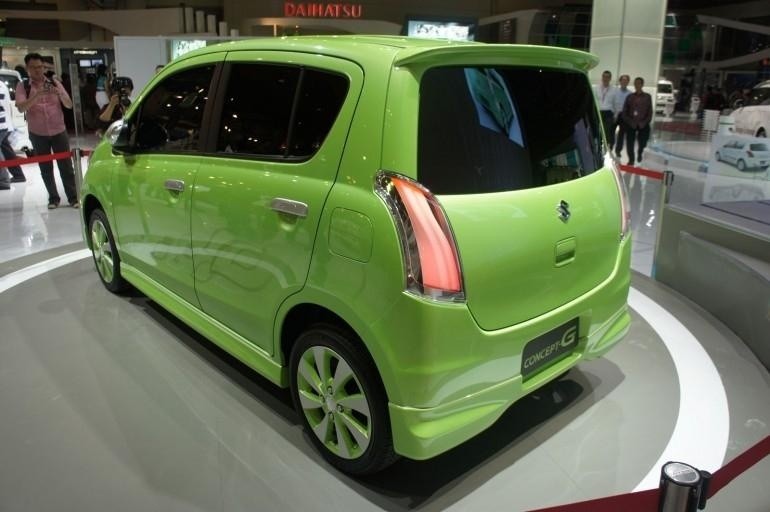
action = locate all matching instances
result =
[113,79,131,107]
[45,79,52,91]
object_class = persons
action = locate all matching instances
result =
[154,65,165,74]
[41,63,112,135]
[612,74,633,157]
[93,76,134,135]
[14,51,81,211]
[592,69,615,152]
[670,79,746,118]
[0,45,27,191]
[621,76,652,166]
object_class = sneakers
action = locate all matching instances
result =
[72,203,80,208]
[637,150,643,162]
[48,201,59,209]
[627,160,634,166]
[615,149,621,158]
[10,176,25,183]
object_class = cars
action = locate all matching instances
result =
[728,80,770,137]
[80,34,632,480]
[716,139,770,171]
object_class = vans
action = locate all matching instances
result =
[0,69,26,127]
[656,80,673,113]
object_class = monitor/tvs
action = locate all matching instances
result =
[405,15,478,43]
